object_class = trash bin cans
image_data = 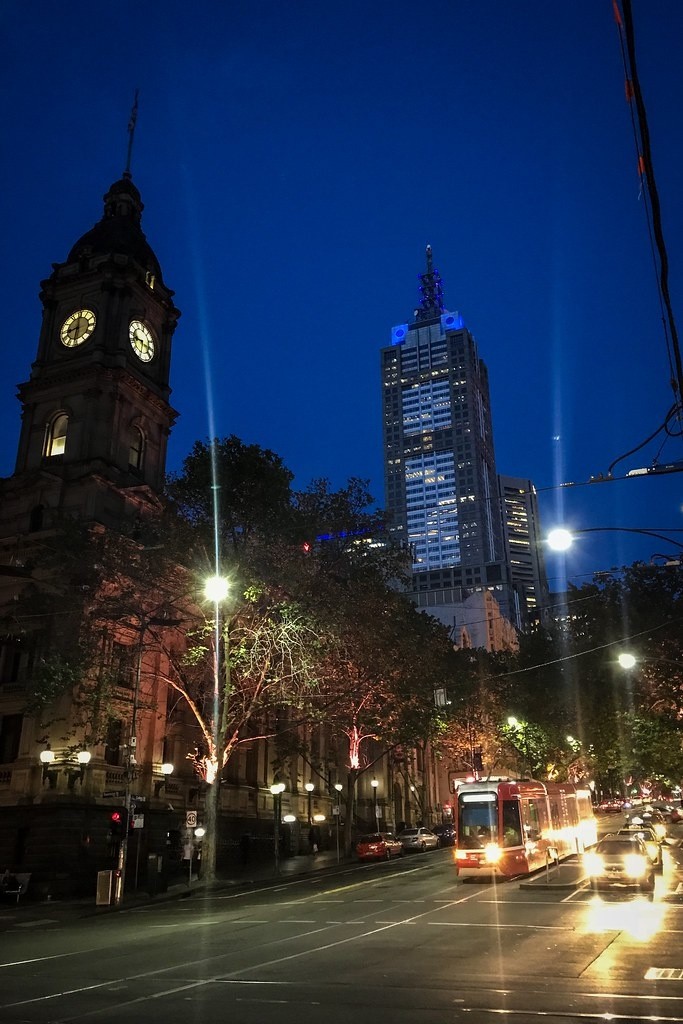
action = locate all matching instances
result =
[95,869,117,905]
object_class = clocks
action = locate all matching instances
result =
[128,320,155,363]
[59,308,96,348]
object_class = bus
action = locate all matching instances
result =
[448,780,597,879]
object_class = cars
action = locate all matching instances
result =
[432,826,456,846]
[397,826,441,853]
[355,832,404,863]
[592,793,683,837]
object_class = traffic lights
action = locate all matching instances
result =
[111,805,128,835]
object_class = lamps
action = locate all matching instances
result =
[151,763,174,800]
[63,751,92,793]
[39,744,62,788]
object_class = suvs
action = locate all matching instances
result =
[589,832,656,890]
[616,827,663,870]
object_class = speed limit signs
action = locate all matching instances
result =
[186,813,197,826]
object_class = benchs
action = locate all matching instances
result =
[0,872,33,903]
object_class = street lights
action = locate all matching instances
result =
[371,780,378,834]
[334,784,342,862]
[305,783,315,825]
[270,783,285,873]
[202,575,230,894]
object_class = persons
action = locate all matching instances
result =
[307,819,320,860]
[182,837,194,879]
[195,840,202,875]
